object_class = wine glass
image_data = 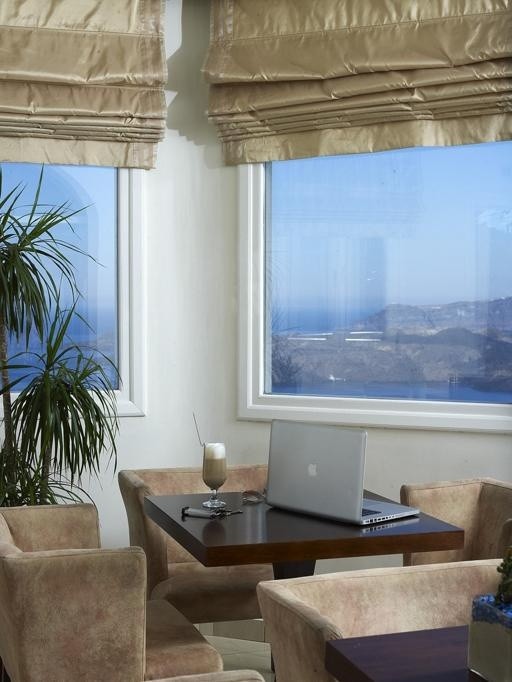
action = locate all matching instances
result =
[201,442,228,508]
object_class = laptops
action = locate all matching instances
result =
[266,417,421,528]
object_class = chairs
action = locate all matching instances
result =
[118,462,273,622]
[256,558,512,682]
[400,476,512,565]
[0,503,263,682]
